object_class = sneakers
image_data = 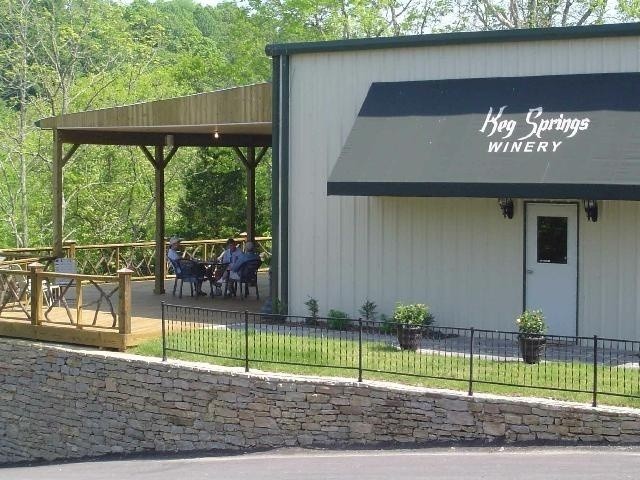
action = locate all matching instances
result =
[194,278,235,297]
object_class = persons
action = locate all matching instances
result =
[217,241,256,296]
[212,238,243,299]
[167,235,222,298]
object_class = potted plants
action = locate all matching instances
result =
[513,307,545,364]
[393,296,429,350]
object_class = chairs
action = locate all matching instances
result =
[0,258,79,309]
[166,253,262,301]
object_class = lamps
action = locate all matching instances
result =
[583,199,597,222]
[497,197,513,219]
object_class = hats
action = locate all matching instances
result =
[168,237,183,245]
